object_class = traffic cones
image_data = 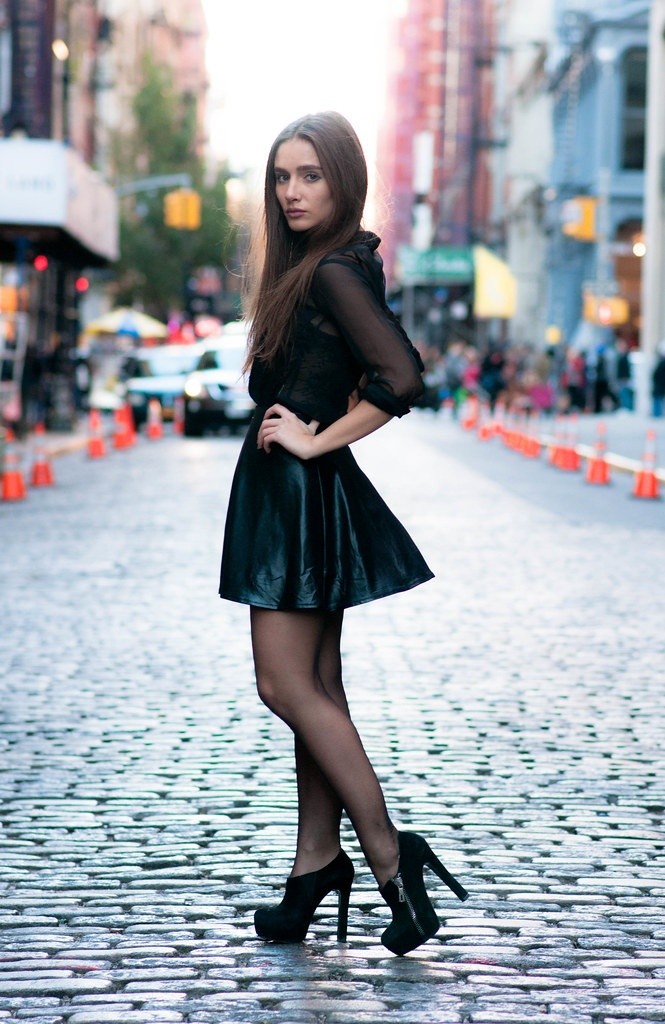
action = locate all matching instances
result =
[0,426,30,504]
[442,393,583,470]
[25,423,55,487]
[83,395,187,457]
[584,419,612,485]
[629,426,658,503]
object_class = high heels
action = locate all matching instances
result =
[254,848,355,943]
[378,831,470,956]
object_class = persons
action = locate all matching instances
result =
[203,108,473,960]
[77,302,665,428]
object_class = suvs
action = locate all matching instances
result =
[86,320,262,436]
